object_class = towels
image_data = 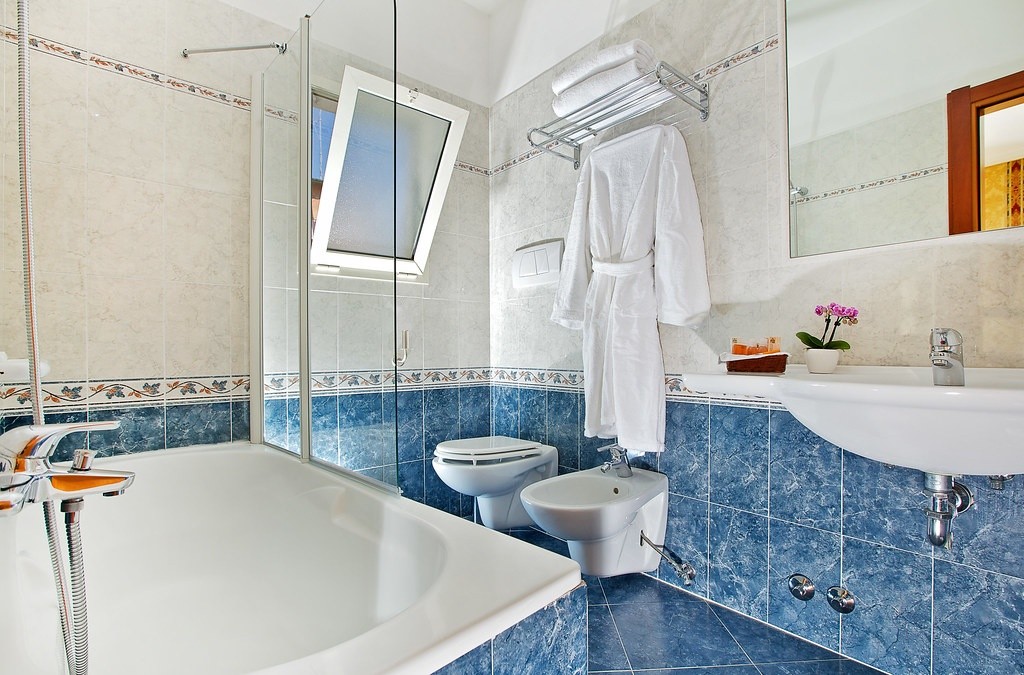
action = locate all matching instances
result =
[551,37,677,134]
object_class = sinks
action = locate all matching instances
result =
[519,464,670,579]
[681,363,1023,479]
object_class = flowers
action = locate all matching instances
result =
[797,303,859,352]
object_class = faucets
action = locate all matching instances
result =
[596,442,634,478]
[1,419,137,516]
[928,326,966,386]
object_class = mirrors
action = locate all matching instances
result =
[787,0,1024,258]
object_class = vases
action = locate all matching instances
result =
[805,349,839,373]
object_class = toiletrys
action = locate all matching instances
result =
[729,335,782,355]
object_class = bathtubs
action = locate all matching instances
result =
[0,441,583,675]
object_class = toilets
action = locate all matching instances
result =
[431,435,559,531]
